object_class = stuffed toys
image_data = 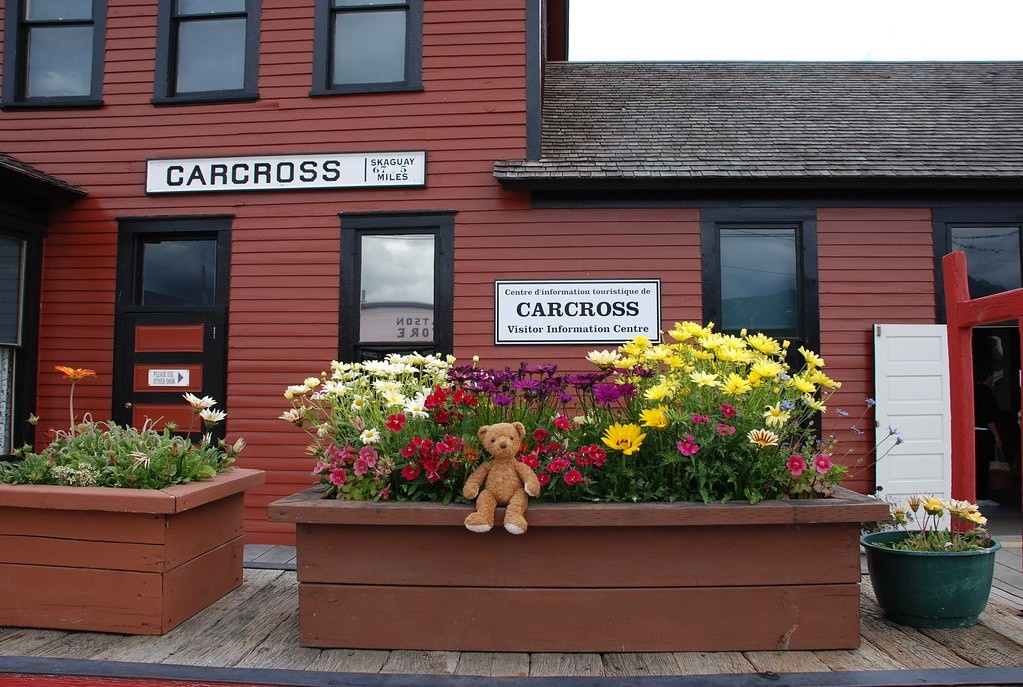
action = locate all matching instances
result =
[463,422,540,534]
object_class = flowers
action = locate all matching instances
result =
[275,314,902,502]
[0,364,246,488]
[860,486,991,551]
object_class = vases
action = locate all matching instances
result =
[861,530,1002,630]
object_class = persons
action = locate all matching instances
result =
[974,365,1003,506]
